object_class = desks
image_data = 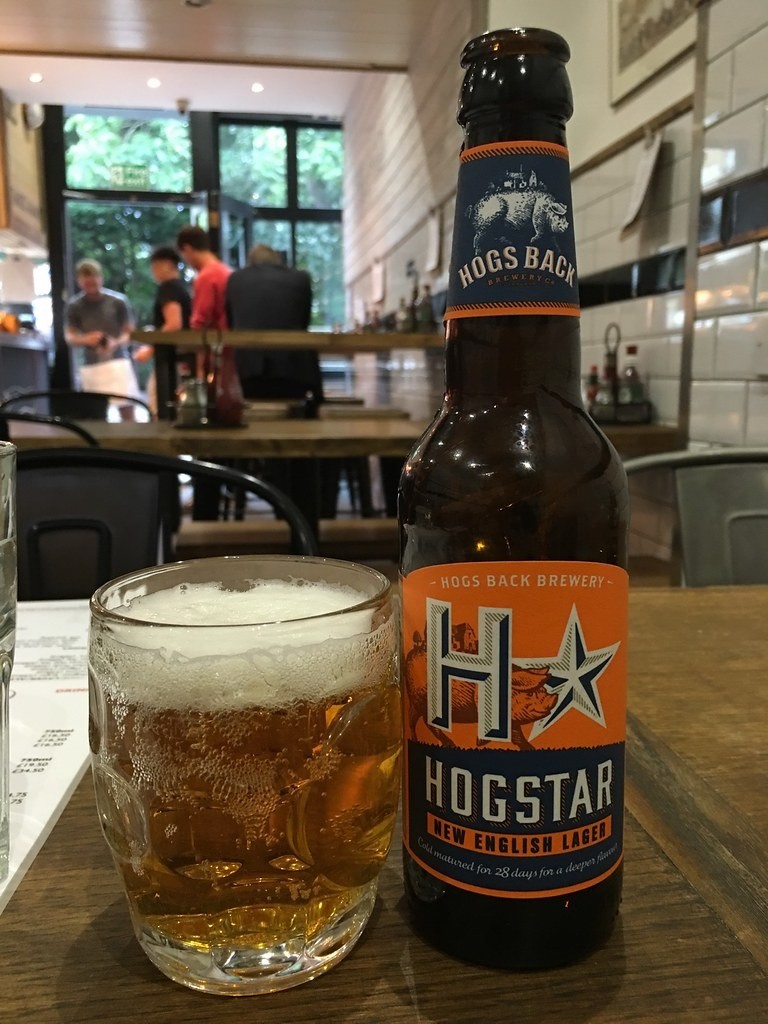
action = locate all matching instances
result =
[130,327,449,422]
[1,386,682,560]
[0,585,767,1023]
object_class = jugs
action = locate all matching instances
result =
[176,379,208,426]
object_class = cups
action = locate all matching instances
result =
[1,441,14,884]
[87,554,395,998]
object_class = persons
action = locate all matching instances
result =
[63,259,139,420]
[176,226,245,521]
[223,243,341,520]
[124,247,193,524]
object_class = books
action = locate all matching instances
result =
[0,597,106,915]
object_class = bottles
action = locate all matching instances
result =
[396,27,629,970]
[586,366,599,403]
[621,344,644,405]
[395,283,433,332]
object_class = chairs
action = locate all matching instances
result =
[12,447,322,603]
[621,447,767,587]
